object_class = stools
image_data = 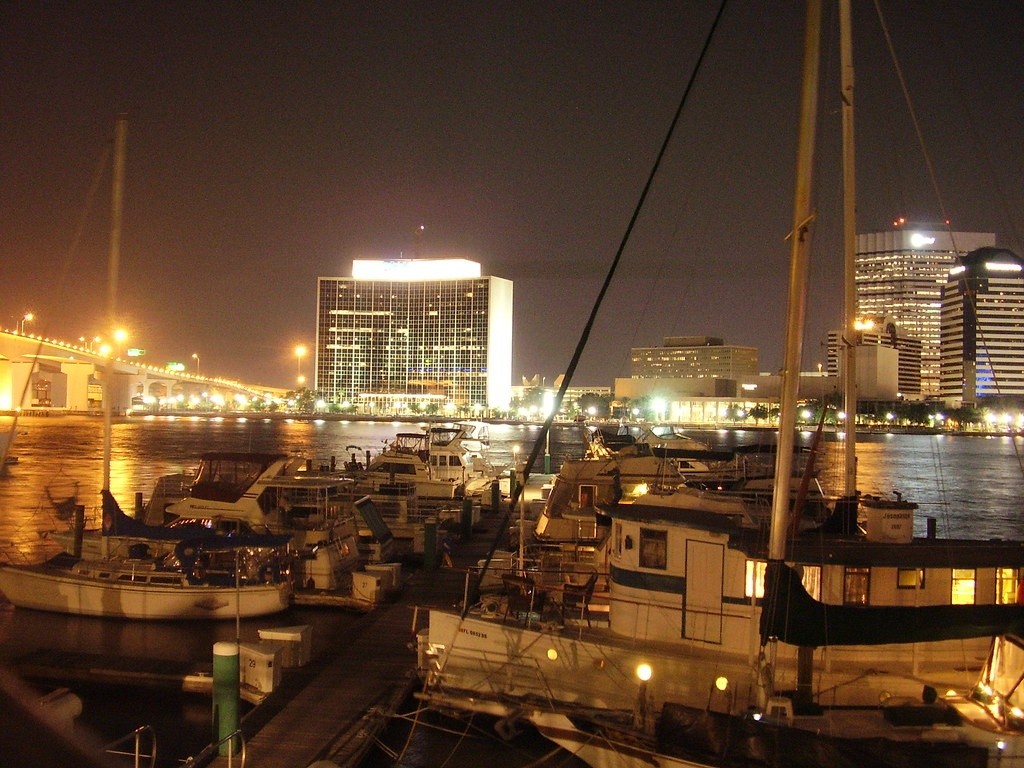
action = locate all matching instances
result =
[767,697,793,727]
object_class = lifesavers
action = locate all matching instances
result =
[173,542,201,566]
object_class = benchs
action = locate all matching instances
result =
[883,705,962,727]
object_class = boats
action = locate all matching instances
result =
[408,0,1024,768]
[0,114,511,622]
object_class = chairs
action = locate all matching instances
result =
[562,572,599,628]
[501,573,547,624]
